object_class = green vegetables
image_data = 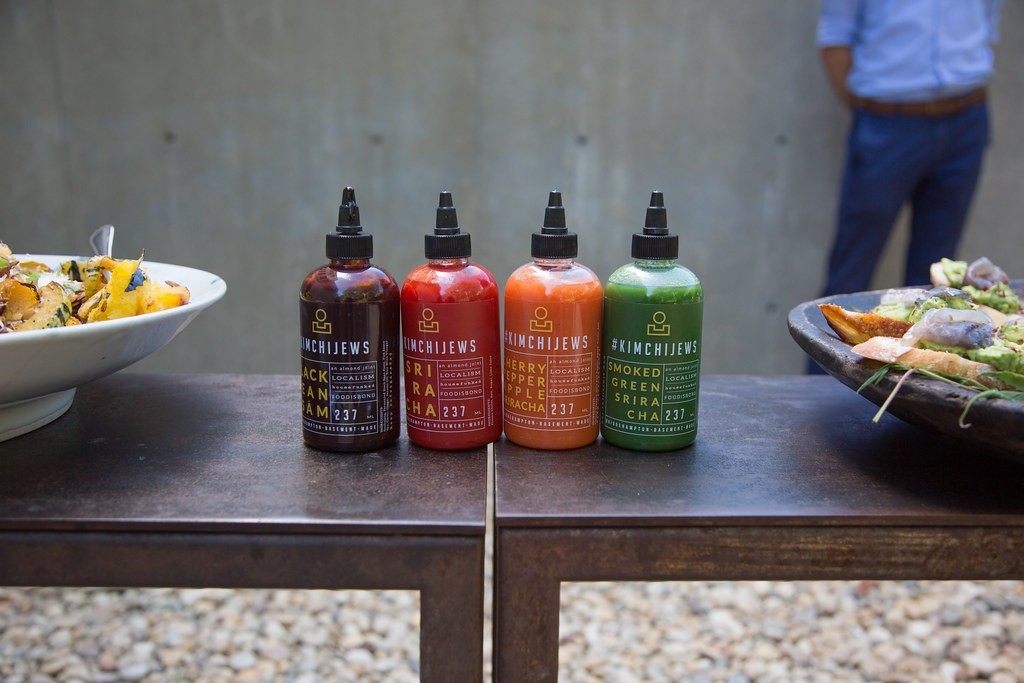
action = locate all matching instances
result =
[856,362,1024,428]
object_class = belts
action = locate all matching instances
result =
[848,87,986,118]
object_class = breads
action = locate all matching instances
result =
[818,303,914,345]
[850,336,1007,389]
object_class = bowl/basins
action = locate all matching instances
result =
[0,253,227,444]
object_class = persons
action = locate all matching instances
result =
[809,1,997,374]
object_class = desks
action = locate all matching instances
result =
[493,377,1024,683]
[0,375,486,683]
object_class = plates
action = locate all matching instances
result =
[786,286,1024,463]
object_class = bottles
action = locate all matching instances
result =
[503,192,602,449]
[402,192,504,451]
[300,188,400,452]
[602,193,705,451]
